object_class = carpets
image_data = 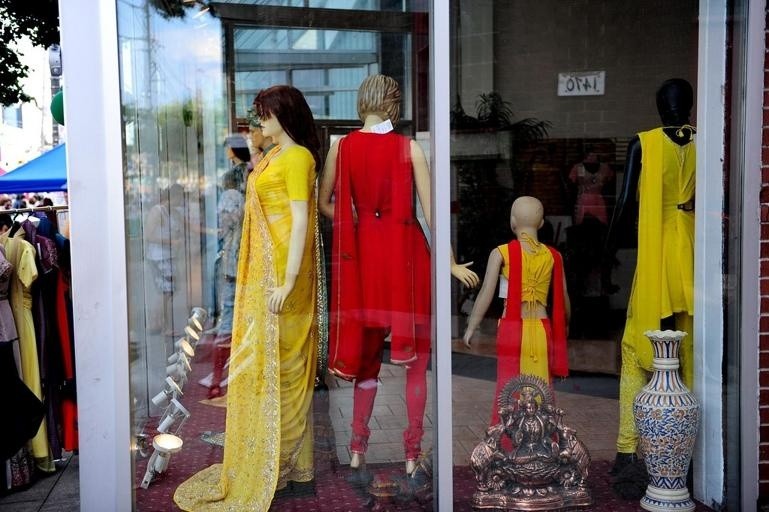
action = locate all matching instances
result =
[137,312,361,512]
[338,457,648,512]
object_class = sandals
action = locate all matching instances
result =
[207,383,221,400]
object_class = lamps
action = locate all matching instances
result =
[138,305,210,496]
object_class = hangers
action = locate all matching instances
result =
[8,205,57,238]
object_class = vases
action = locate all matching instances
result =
[633,328,701,512]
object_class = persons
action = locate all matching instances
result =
[172,85,326,511]
[508,389,555,467]
[0,180,208,340]
[208,109,276,339]
[465,192,572,451]
[320,73,479,475]
[586,76,697,476]
